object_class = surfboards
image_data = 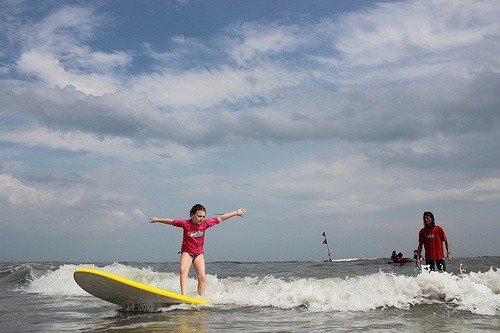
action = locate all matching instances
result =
[73,267,210,314]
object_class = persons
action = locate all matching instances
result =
[416,211,452,272]
[388,250,424,263]
[150,204,247,296]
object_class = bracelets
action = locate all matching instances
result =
[447,253,451,255]
[237,210,239,216]
